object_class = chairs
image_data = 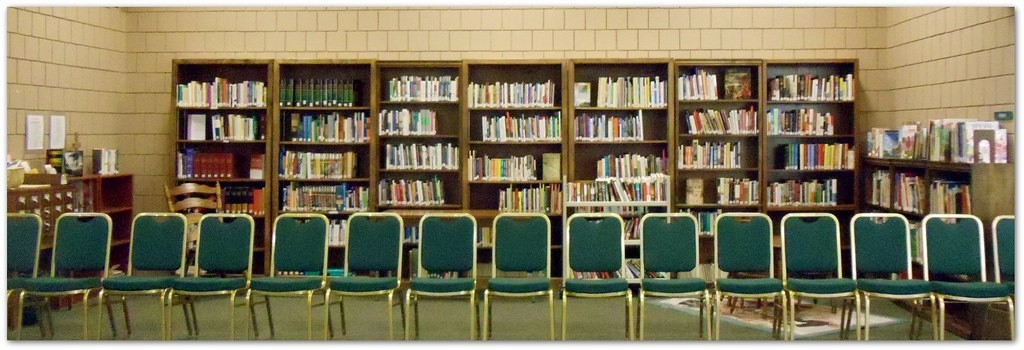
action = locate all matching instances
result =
[98,212,192,341]
[777,212,862,342]
[560,212,635,340]
[324,212,404,340]
[405,213,478,340]
[844,212,938,341]
[992,215,1015,284]
[7,213,47,338]
[921,212,1014,342]
[163,180,224,249]
[482,213,556,341]
[169,213,258,341]
[638,212,713,341]
[246,213,331,340]
[17,213,116,341]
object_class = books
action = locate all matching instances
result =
[277,78,371,277]
[379,75,461,279]
[45,149,63,174]
[175,77,267,277]
[870,218,969,281]
[92,149,119,175]
[467,79,563,278]
[867,118,1008,165]
[767,73,856,280]
[64,151,83,177]
[568,76,671,280]
[871,169,972,225]
[677,68,758,284]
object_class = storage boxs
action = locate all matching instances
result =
[23,173,61,185]
[974,128,1007,164]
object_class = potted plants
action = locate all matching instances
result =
[64,131,85,177]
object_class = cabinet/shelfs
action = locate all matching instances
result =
[169,58,976,341]
[66,172,136,268]
[7,183,75,249]
[862,157,1015,339]
